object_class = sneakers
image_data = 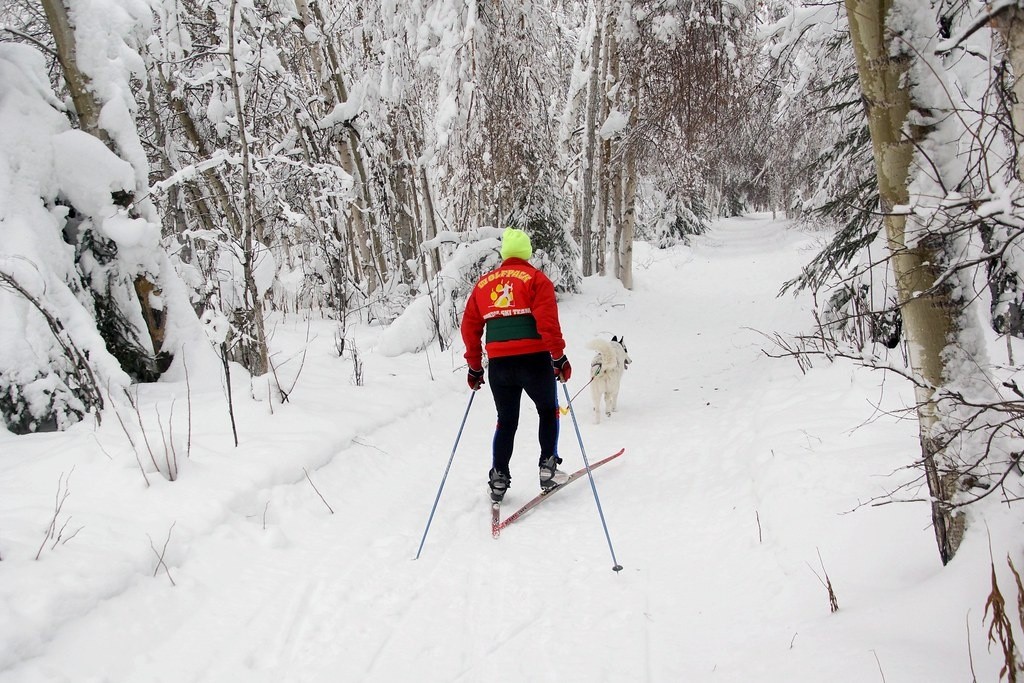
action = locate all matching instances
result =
[539,455,569,489]
[487,467,511,501]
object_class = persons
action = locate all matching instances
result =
[461,228,572,502]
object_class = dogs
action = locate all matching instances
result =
[587,335,632,425]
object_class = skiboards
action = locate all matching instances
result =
[491,444,625,541]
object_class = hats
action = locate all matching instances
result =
[500,227,532,260]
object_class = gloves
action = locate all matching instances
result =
[551,354,572,382]
[468,366,485,390]
